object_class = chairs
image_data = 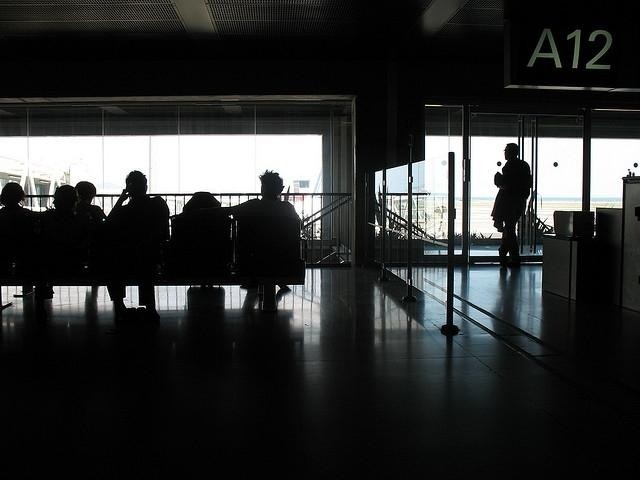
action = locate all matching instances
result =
[0,206,303,316]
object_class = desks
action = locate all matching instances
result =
[543,232,598,299]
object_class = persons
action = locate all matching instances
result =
[493,141,532,271]
[105,171,169,319]
[183,169,300,312]
[0,182,105,296]
[492,269,521,338]
